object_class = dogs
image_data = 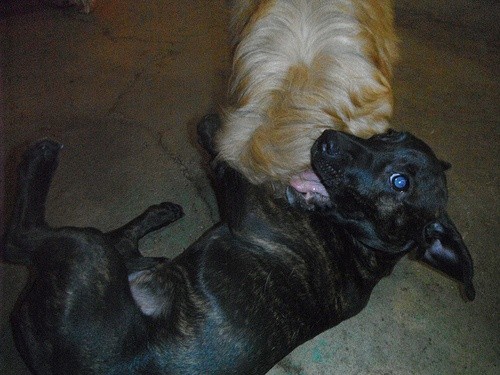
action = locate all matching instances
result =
[0,112,477,375]
[212,0,403,186]
